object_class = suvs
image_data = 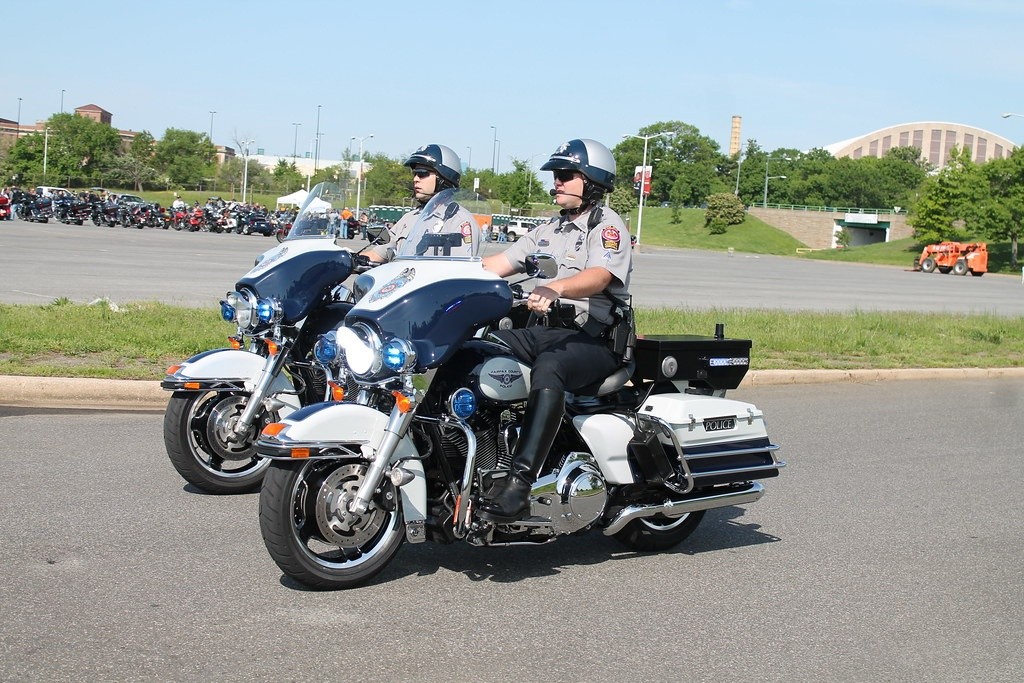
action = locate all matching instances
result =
[506,220,538,242]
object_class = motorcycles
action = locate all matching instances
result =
[161,183,787,588]
[0,183,353,242]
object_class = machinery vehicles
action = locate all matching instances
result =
[920,240,988,277]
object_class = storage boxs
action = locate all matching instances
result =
[640,393,780,486]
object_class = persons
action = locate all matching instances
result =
[215,197,268,215]
[360,213,368,240]
[44,188,66,221]
[357,143,481,260]
[634,172,642,187]
[173,196,185,208]
[72,189,115,203]
[1,186,37,221]
[328,207,353,239]
[475,138,633,522]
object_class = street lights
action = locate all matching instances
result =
[489,125,503,178]
[60,89,66,121]
[18,97,23,139]
[207,109,217,141]
[622,132,674,244]
[44,127,52,176]
[292,103,375,197]
[467,145,473,168]
[762,158,793,209]
[240,138,258,203]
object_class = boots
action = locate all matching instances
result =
[476,388,566,523]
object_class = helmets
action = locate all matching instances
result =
[539,138,616,193]
[402,144,462,189]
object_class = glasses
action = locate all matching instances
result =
[554,170,591,182]
[412,167,435,179]
